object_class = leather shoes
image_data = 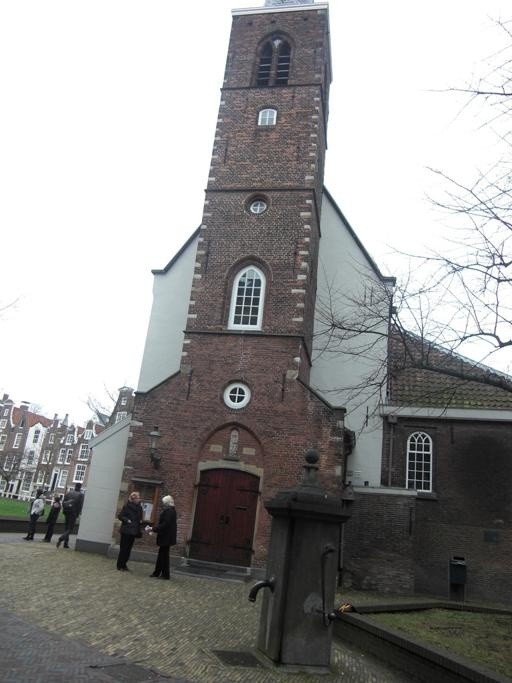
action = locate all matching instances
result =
[150,573,170,579]
[117,562,128,571]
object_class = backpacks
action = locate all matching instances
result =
[62,499,81,516]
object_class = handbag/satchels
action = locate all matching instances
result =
[137,531,142,538]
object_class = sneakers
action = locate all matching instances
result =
[23,536,70,548]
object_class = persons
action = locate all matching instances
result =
[146,494,177,580]
[116,491,144,572]
[22,488,62,543]
[56,483,84,549]
